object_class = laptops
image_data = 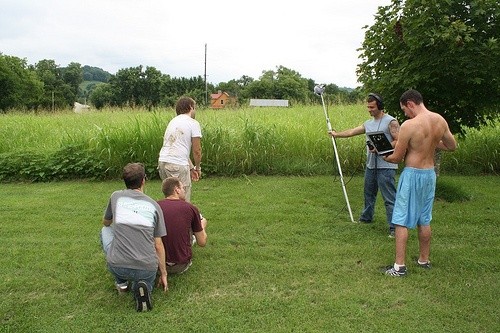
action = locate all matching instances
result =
[366,130,396,156]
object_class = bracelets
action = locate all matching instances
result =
[194,166,201,171]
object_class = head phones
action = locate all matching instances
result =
[368,92,385,110]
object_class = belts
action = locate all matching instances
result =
[165,263,178,267]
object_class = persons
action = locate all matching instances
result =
[101,162,169,313]
[158,97,203,247]
[329,92,401,239]
[382,90,456,278]
[156,177,207,273]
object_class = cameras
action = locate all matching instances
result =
[366,141,374,150]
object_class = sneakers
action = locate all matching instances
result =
[136,282,152,312]
[115,280,129,294]
[382,263,407,278]
[414,259,433,268]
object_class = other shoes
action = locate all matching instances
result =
[388,230,395,238]
[191,235,196,247]
[179,261,193,275]
